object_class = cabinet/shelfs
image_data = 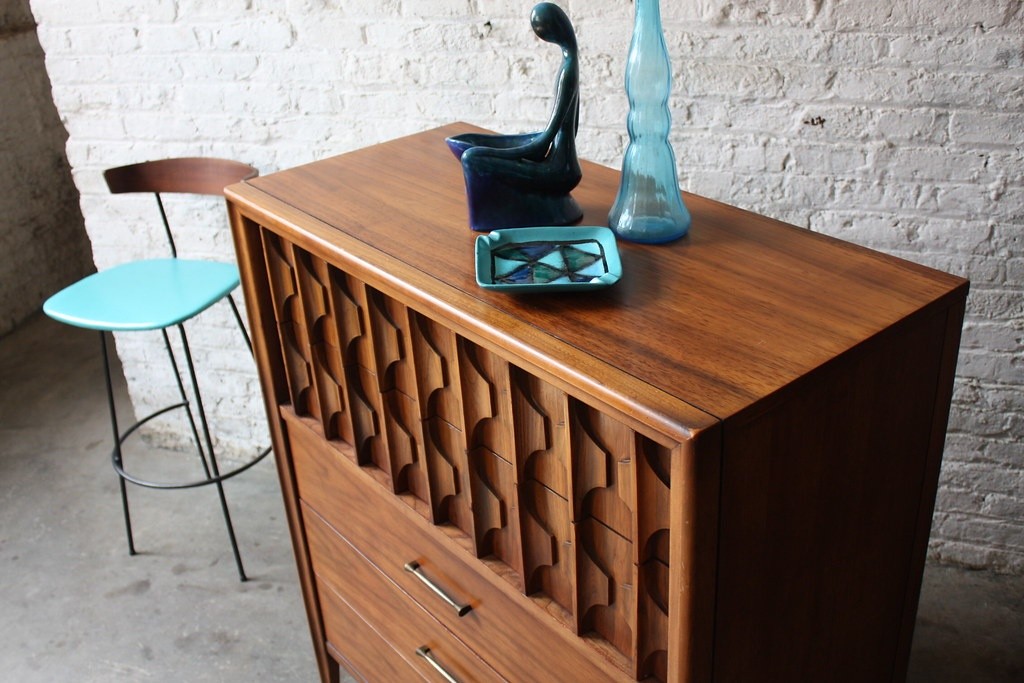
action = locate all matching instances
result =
[223,122,971,683]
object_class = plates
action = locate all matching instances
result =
[475,225,623,291]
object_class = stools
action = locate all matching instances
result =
[44,156,274,582]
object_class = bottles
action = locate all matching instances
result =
[608,0,691,243]
